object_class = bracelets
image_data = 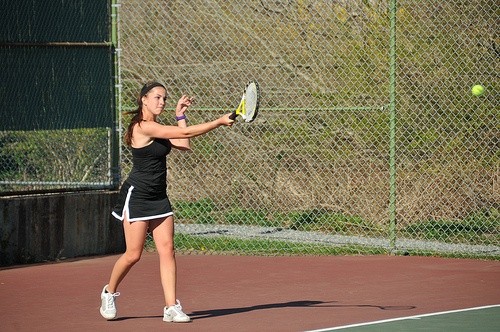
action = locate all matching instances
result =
[176,116,185,121]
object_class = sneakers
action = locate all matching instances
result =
[100,284,120,319]
[163,299,191,322]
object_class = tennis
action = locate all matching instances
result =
[472,85,485,96]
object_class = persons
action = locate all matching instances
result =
[99,82,235,323]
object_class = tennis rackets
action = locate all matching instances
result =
[229,79,260,123]
